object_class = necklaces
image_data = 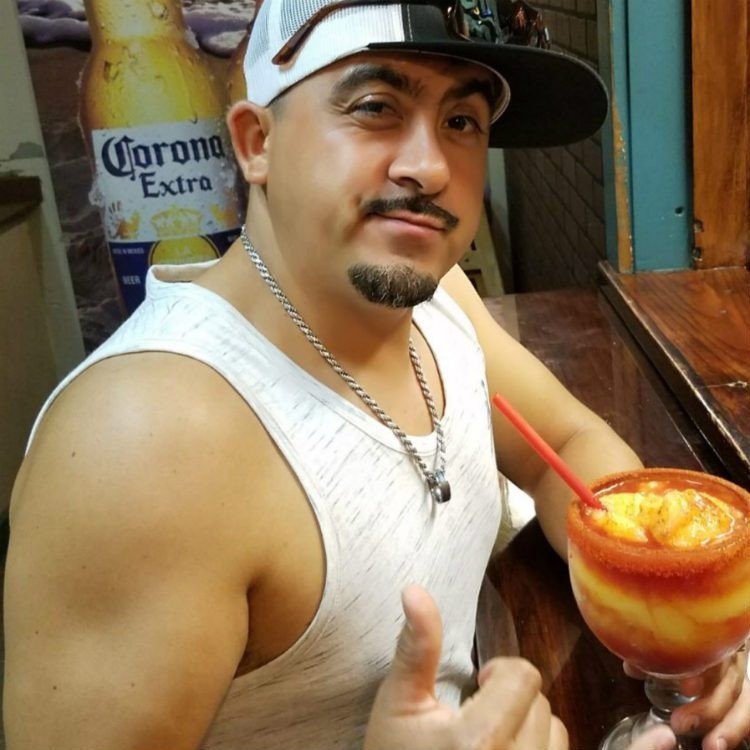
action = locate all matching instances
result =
[242,222,452,503]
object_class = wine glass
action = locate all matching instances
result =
[567,467,749,750]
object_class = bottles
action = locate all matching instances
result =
[74,0,266,325]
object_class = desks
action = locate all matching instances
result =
[470,263,750,750]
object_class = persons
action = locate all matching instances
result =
[5,0,750,750]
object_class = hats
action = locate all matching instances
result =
[243,0,609,151]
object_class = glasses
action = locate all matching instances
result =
[271,0,552,67]
